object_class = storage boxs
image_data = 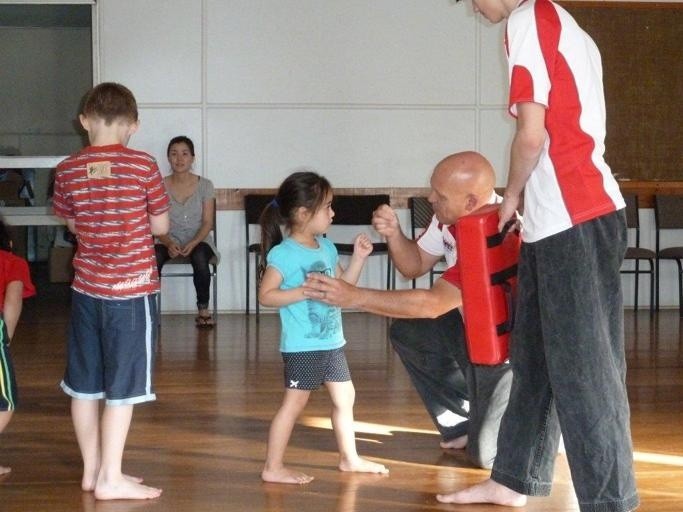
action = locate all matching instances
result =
[48,246,75,283]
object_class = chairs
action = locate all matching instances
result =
[158,197,218,325]
[324,194,396,292]
[655,195,683,319]
[244,193,280,326]
[620,192,656,319]
[409,194,447,289]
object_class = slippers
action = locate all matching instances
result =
[196,314,215,328]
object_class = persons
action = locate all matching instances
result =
[428,0,645,511]
[51,80,171,502]
[299,149,523,470]
[0,216,38,477]
[253,169,391,484]
[153,134,217,328]
